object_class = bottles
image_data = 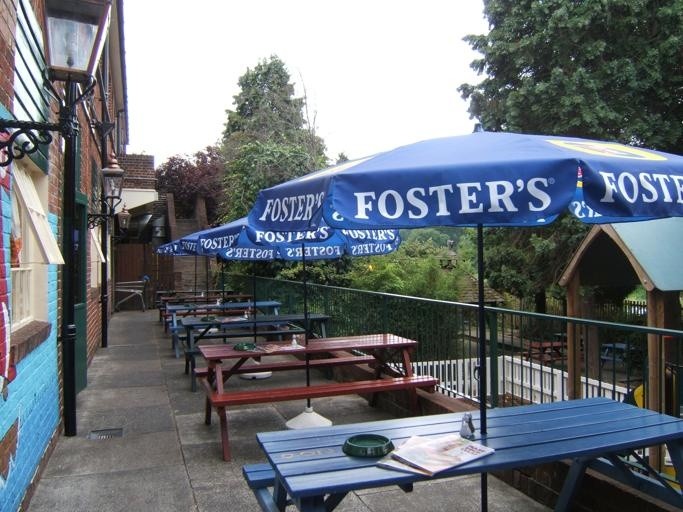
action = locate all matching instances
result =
[459,414,475,439]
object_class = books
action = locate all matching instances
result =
[376,435,440,478]
[390,430,494,474]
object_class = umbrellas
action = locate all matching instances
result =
[196,213,399,405]
[153,227,216,321]
[226,128,682,512]
[178,215,294,342]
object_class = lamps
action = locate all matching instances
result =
[87,150,126,228]
[0,0,114,171]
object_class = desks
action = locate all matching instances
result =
[525,341,567,365]
[197,329,417,463]
[159,294,255,322]
[255,396,683,512]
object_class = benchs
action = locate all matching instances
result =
[522,351,568,366]
[156,300,332,393]
[209,372,439,408]
[194,354,375,376]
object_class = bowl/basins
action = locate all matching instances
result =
[201,316,216,322]
[342,433,395,457]
[232,342,255,351]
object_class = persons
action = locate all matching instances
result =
[214,291,223,305]
